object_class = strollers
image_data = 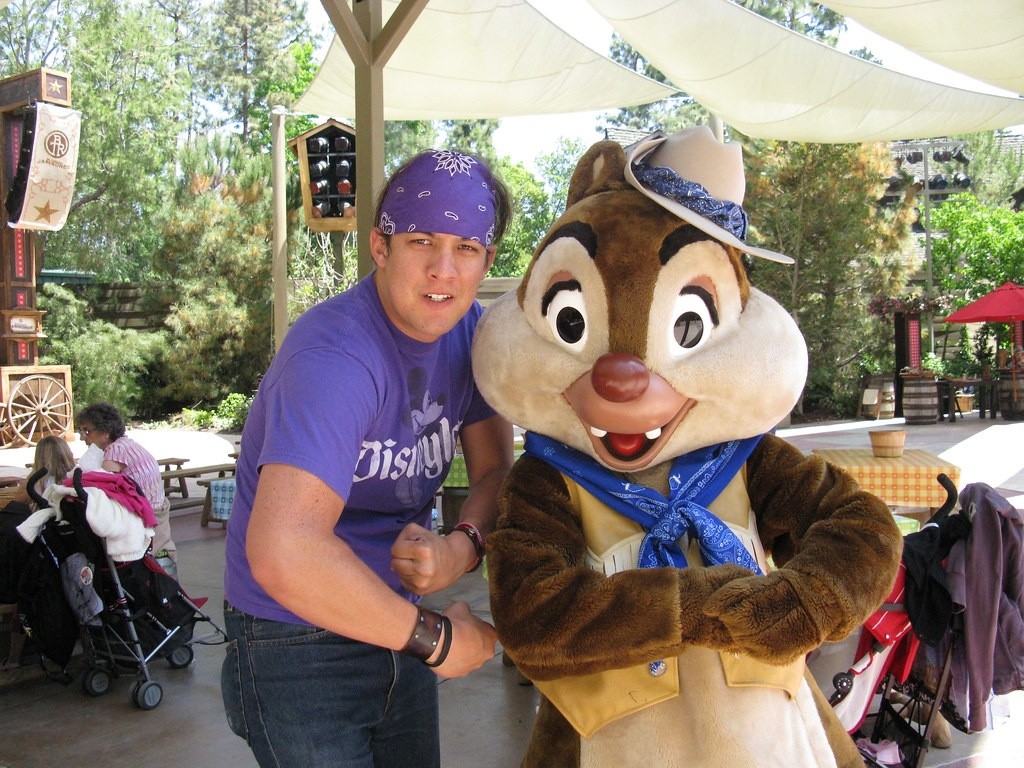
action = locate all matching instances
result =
[1,467,227,711]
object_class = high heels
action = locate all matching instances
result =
[19,655,34,665]
[46,670,73,684]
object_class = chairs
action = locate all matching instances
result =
[938,378,963,418]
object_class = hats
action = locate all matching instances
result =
[622,124,795,265]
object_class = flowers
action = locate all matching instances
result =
[869,291,952,324]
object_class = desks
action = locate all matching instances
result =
[948,379,999,422]
[810,448,957,514]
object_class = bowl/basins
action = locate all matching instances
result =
[869,430,906,458]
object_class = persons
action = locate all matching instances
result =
[75,406,178,563]
[12,437,75,513]
[221,150,516,768]
[1009,347,1024,367]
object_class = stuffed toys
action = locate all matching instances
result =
[472,126,904,768]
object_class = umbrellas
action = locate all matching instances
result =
[945,282,1024,402]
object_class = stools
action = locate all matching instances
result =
[0,604,30,670]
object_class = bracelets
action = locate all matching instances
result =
[450,523,485,574]
[396,604,452,668]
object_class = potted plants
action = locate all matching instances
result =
[973,344,994,379]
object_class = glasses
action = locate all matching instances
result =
[78,427,99,436]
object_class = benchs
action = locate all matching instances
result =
[156,453,242,530]
